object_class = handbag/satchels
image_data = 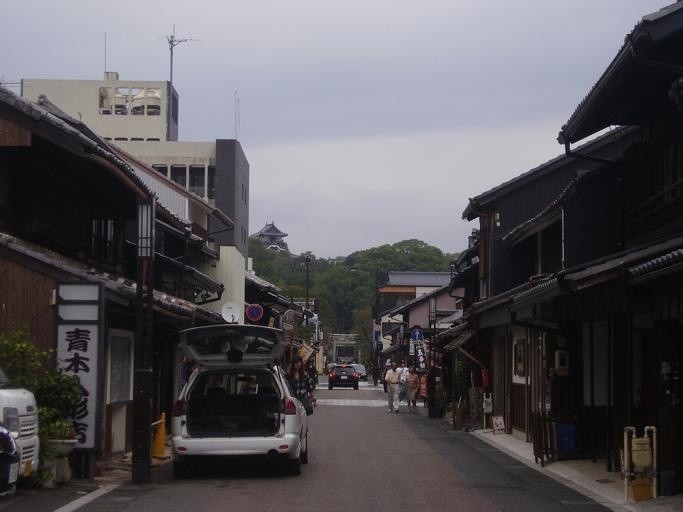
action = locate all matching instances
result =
[301,399,313,416]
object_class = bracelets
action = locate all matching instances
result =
[312,398,316,402]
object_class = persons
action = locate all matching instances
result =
[395,360,409,404]
[404,366,420,413]
[384,362,401,413]
[286,355,318,416]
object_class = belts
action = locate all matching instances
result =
[391,382,399,384]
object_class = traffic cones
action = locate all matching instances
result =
[150,411,168,459]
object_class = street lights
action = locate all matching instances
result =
[350,266,380,316]
[132,198,156,482]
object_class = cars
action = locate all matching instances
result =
[170,323,308,478]
[329,364,367,391]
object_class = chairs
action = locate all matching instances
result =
[206,387,275,394]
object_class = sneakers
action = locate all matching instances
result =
[387,400,419,414]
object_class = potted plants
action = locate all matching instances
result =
[0,325,87,488]
